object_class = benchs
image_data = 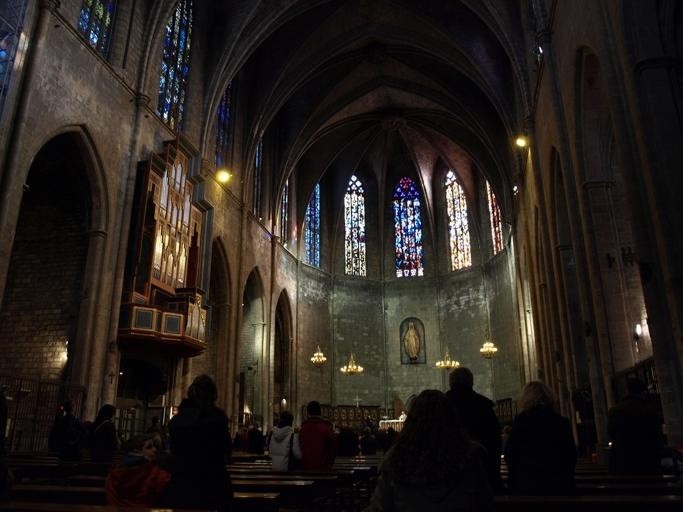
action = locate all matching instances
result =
[0,449,683,512]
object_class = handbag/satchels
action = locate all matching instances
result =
[287,455,300,471]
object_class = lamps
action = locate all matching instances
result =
[480,342,498,359]
[435,351,460,370]
[634,324,642,352]
[310,344,327,369]
[340,352,364,376]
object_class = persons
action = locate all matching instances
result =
[101,432,173,510]
[337,427,358,456]
[397,410,407,420]
[500,425,510,449]
[442,365,498,495]
[153,373,235,512]
[607,378,665,473]
[363,426,399,456]
[299,400,337,475]
[361,388,499,512]
[238,423,264,454]
[501,377,580,497]
[54,400,117,455]
[270,411,300,473]
